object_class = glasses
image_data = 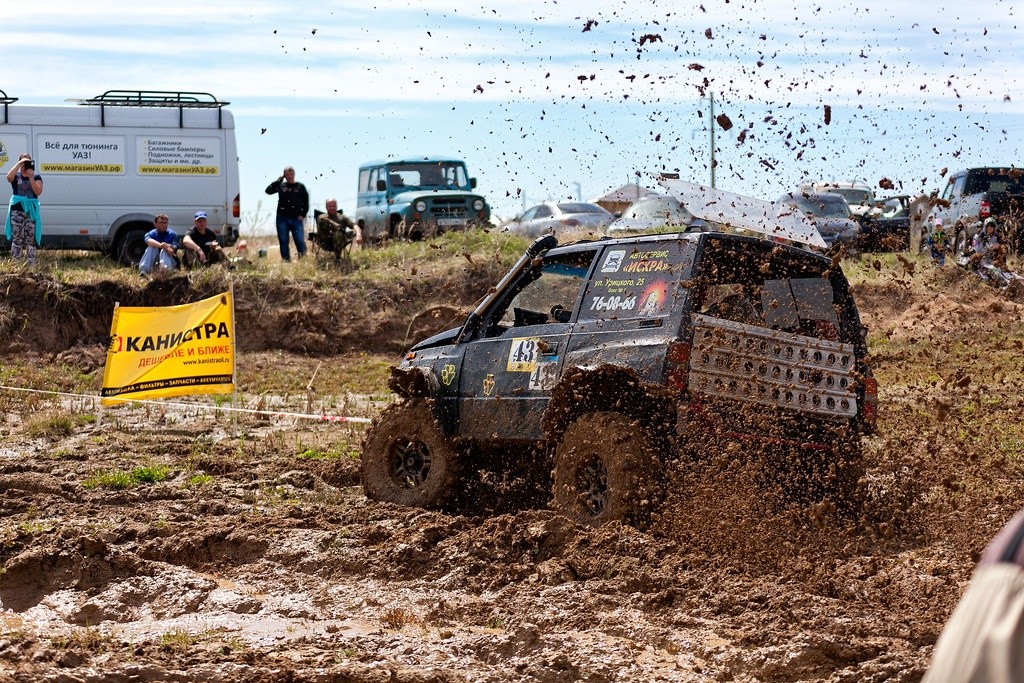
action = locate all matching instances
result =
[159,222,169,226]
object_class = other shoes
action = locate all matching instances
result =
[334,257,340,267]
[345,230,356,242]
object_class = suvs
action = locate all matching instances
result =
[356,156,491,243]
[360,220,879,531]
[771,181,913,258]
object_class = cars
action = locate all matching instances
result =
[503,202,614,238]
[605,196,697,236]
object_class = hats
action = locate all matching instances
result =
[194,211,208,221]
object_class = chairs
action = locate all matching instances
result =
[377,171,441,191]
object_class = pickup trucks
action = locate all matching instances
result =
[917,166,1024,257]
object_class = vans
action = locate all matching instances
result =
[0,88,240,270]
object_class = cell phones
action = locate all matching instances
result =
[25,160,35,172]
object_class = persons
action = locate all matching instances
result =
[139,214,180,276]
[7,153,43,262]
[177,211,236,271]
[927,217,1017,287]
[315,199,363,262]
[265,166,312,262]
